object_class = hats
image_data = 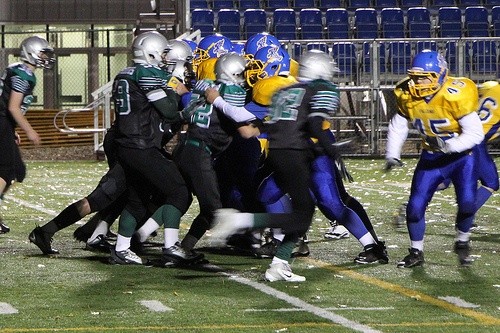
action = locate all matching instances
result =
[407,51,447,98]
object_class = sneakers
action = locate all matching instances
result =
[29,226,59,254]
[324,221,349,239]
[133,233,143,253]
[162,243,209,264]
[86,231,118,246]
[74,227,112,252]
[397,247,424,270]
[354,244,389,264]
[203,208,311,258]
[108,248,153,266]
[359,242,387,258]
[264,265,306,283]
[454,242,472,268]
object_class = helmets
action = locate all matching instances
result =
[131,31,171,71]
[168,32,336,84]
[19,37,56,70]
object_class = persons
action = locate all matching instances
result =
[393,78,500,233]
[380,50,485,268]
[0,36,56,234]
[28,30,389,282]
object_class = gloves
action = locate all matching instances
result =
[418,133,445,150]
[181,96,206,120]
[384,159,401,171]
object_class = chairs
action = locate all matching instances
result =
[190,0,500,77]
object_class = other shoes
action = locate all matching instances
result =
[0,222,9,233]
